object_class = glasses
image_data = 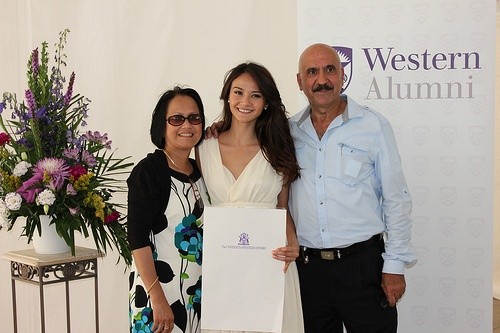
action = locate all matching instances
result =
[188,177,204,211]
[166,113,202,125]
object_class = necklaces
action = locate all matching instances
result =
[163,149,182,171]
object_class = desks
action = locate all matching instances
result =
[0,245,106,333]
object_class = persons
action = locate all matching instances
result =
[215,44,419,333]
[194,63,305,333]
[123,87,211,333]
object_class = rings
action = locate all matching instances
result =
[164,326,169,329]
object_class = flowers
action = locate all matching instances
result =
[0,28,135,275]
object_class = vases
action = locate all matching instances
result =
[32,214,70,255]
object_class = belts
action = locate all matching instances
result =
[298,234,383,260]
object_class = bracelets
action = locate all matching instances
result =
[147,276,159,292]
